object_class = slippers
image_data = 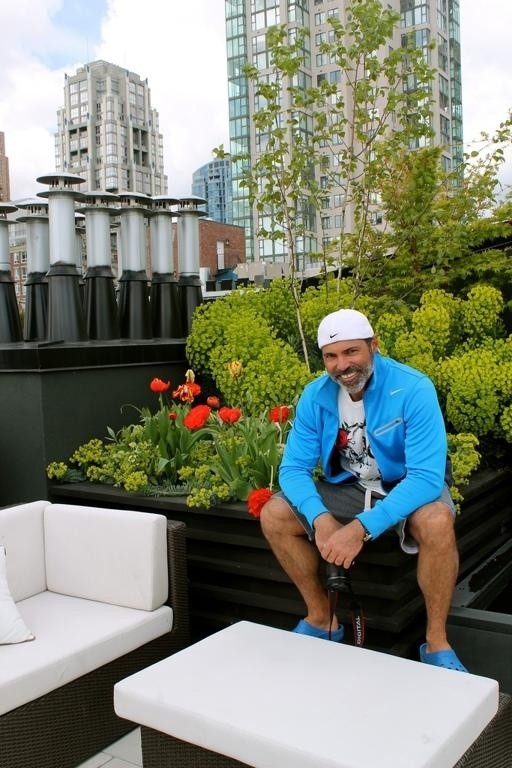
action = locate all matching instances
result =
[419,643,470,675]
[291,618,345,641]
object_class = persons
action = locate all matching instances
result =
[258,308,473,673]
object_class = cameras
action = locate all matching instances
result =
[319,556,357,593]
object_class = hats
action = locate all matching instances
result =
[317,309,375,350]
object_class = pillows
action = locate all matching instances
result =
[1,546,35,645]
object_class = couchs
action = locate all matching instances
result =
[1,497,191,767]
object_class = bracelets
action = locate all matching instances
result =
[363,526,372,542]
